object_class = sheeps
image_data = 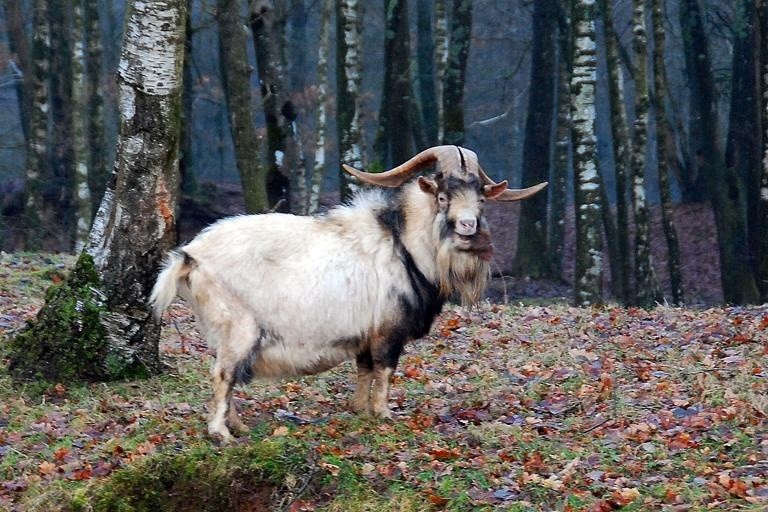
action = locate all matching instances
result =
[147,145,550,444]
